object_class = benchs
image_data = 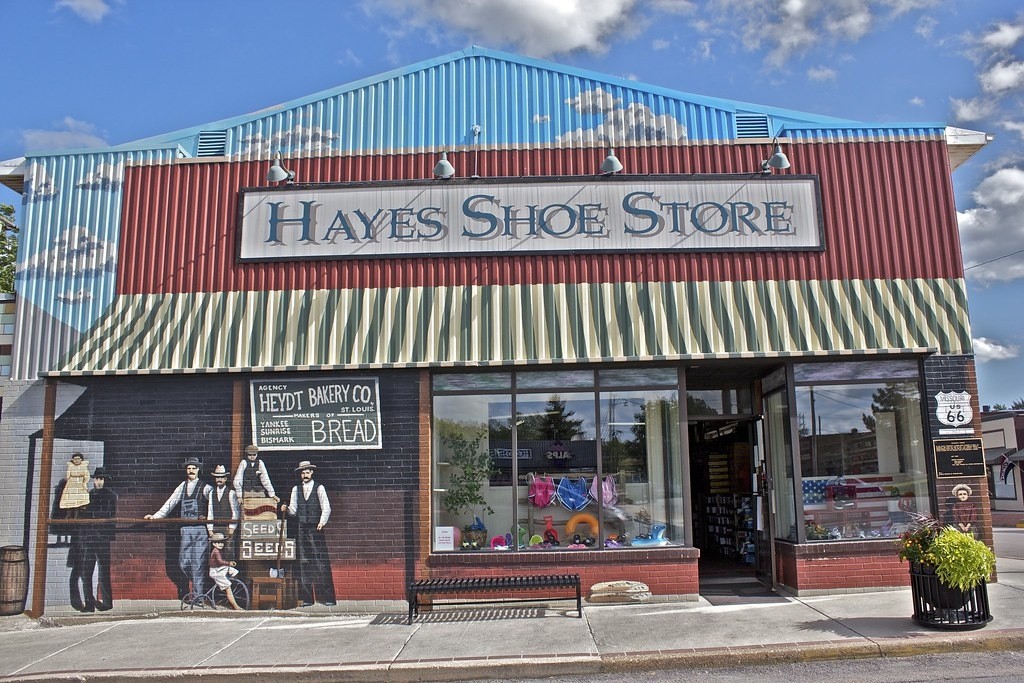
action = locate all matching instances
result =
[408,573,582,625]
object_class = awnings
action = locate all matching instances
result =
[984,448,1017,465]
[1009,448,1024,461]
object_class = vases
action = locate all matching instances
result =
[912,562,973,611]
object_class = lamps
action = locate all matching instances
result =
[760,137,791,169]
[266,150,296,182]
[432,152,455,176]
[601,148,624,172]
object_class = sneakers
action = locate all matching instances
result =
[870,531,881,538]
[841,498,854,506]
[832,495,843,510]
[859,531,865,538]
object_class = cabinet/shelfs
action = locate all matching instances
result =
[816,411,901,476]
[690,424,754,560]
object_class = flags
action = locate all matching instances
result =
[1000,459,1015,486]
[802,478,894,531]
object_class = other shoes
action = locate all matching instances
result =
[882,525,889,537]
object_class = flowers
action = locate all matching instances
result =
[894,524,997,592]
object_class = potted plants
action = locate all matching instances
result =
[440,430,500,548]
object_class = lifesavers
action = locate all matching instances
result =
[565,513,599,536]
